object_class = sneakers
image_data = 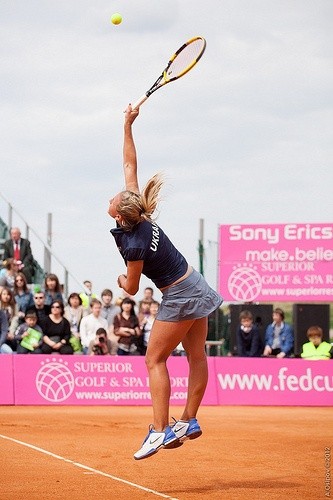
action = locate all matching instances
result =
[133,423,179,461]
[162,416,203,449]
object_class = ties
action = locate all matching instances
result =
[14,241,21,261]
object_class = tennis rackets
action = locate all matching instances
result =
[132,36,206,111]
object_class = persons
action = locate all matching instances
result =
[108,104,223,460]
[0,228,333,360]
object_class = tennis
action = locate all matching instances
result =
[111,13,122,25]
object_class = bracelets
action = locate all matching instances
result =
[117,274,128,288]
[60,341,64,345]
